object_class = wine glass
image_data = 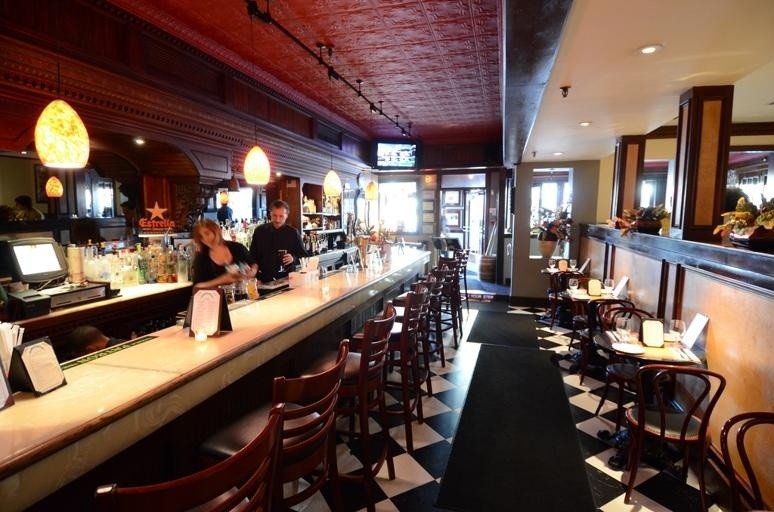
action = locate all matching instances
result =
[568,277,580,293]
[569,259,577,271]
[614,317,631,341]
[669,319,685,347]
[603,278,614,297]
[547,259,556,271]
[277,249,289,273]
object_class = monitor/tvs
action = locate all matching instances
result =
[6,237,69,282]
[377,143,416,169]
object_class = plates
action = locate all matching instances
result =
[611,341,645,355]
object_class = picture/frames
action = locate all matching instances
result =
[420,188,435,236]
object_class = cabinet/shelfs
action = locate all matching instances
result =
[280,178,349,245]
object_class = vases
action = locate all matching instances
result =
[630,220,663,233]
[728,226,774,253]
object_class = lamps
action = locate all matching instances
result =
[322,70,342,200]
[363,102,381,203]
[219,187,229,206]
[41,167,65,201]
[239,6,272,189]
[28,0,89,175]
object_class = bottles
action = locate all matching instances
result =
[303,234,323,255]
[219,275,259,303]
[86,231,189,284]
[217,216,267,251]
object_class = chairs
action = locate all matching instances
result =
[95,404,284,511]
[283,302,396,512]
[348,249,469,456]
[183,338,350,512]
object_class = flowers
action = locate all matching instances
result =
[608,203,670,234]
[713,195,774,236]
[530,211,574,241]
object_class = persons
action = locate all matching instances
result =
[193,219,258,289]
[15,195,45,221]
[217,202,233,224]
[249,200,309,286]
[121,200,137,231]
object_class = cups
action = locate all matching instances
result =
[66,245,84,282]
